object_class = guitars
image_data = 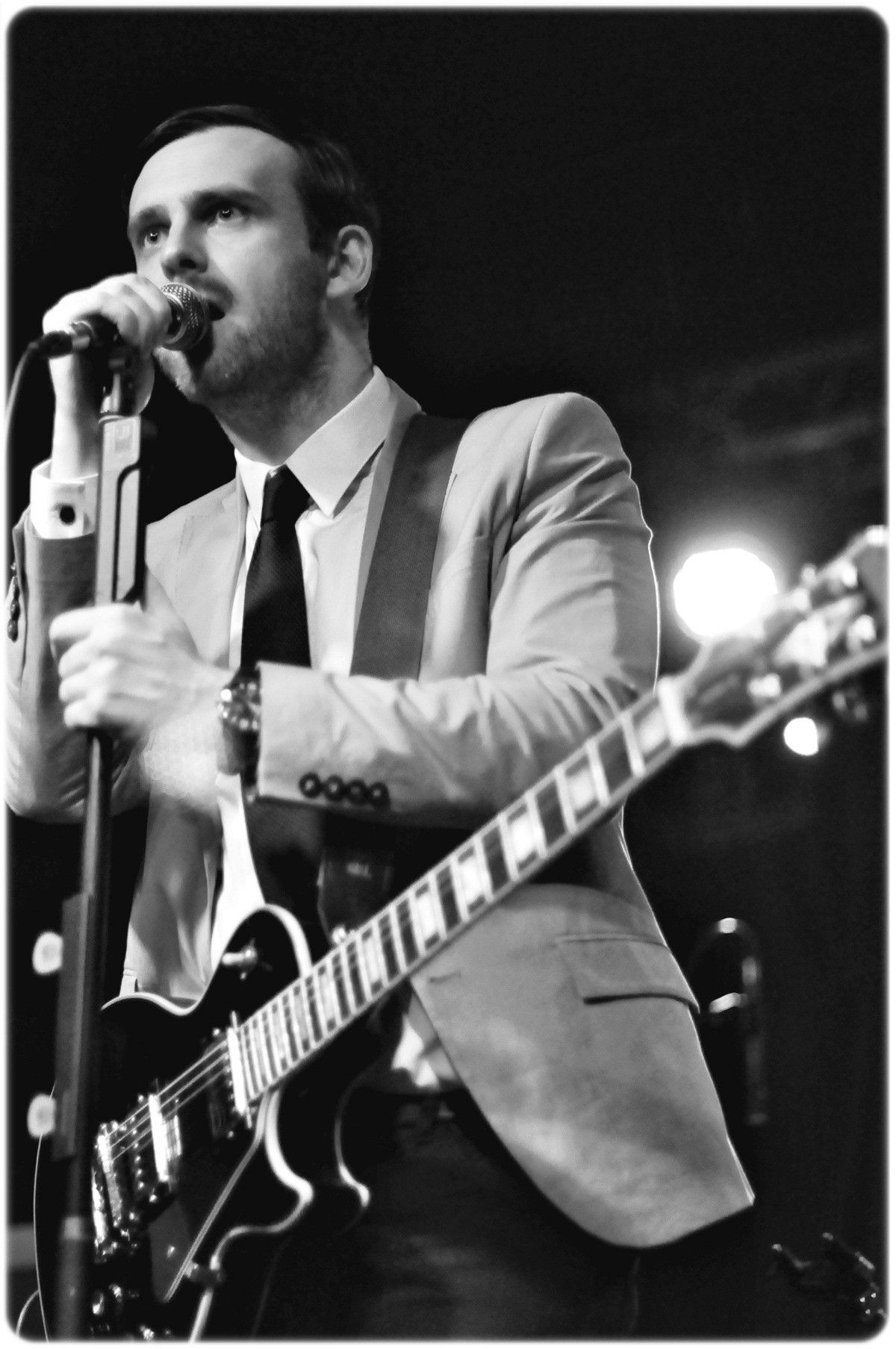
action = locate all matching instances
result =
[33,524,887,1342]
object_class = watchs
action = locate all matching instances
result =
[221,668,259,775]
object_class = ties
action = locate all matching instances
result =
[236,468,328,953]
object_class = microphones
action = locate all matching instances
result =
[29,282,210,360]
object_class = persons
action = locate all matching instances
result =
[5,105,756,1341]
